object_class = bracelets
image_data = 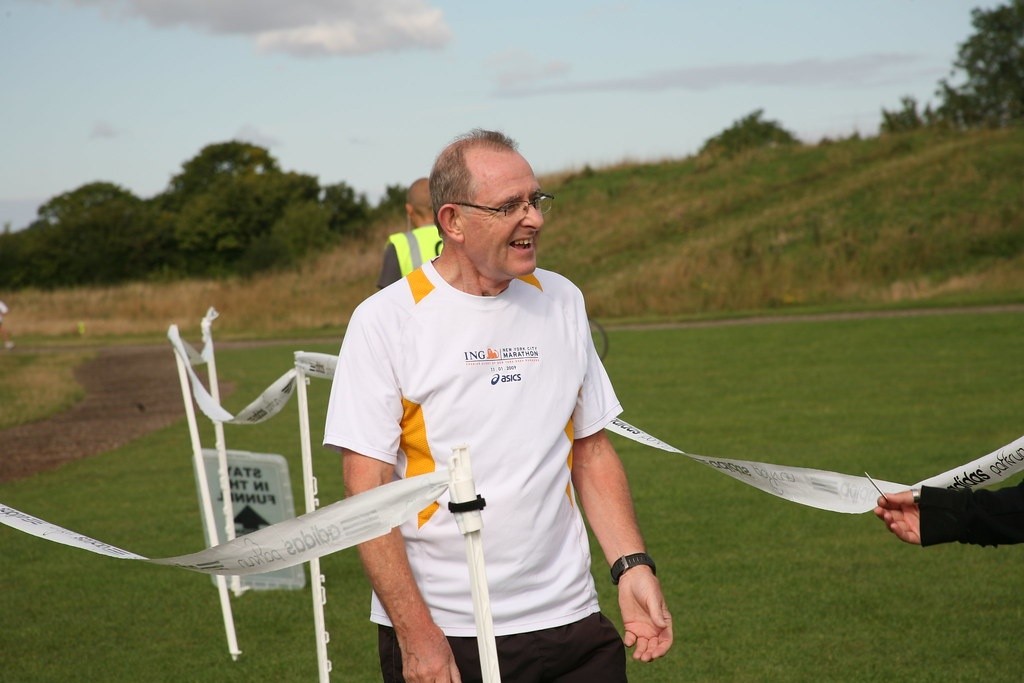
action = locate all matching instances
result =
[609,551,657,585]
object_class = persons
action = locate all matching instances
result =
[323,128,671,683]
[377,178,445,289]
[875,476,1024,548]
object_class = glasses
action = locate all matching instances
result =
[449,193,555,218]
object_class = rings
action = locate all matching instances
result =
[911,489,920,504]
